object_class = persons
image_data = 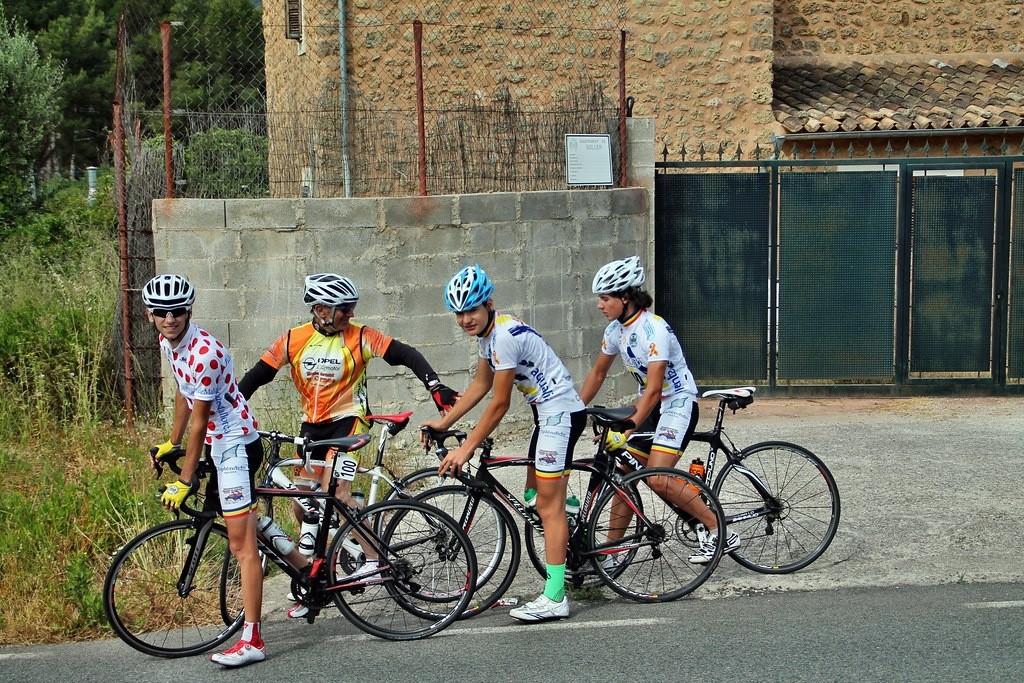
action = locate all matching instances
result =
[578,255,741,572]
[236,273,464,618]
[141,274,309,667]
[417,262,588,621]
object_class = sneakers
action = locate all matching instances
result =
[286,586,307,601]
[208,640,266,668]
[564,554,614,586]
[687,532,741,564]
[509,594,570,624]
[350,565,381,581]
[288,602,309,618]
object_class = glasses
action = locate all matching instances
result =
[335,302,356,313]
[150,307,187,319]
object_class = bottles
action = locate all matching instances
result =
[298,510,319,555]
[258,516,295,555]
[565,496,580,527]
[351,488,364,508]
[671,478,690,487]
[308,480,343,522]
[689,458,704,495]
[524,488,537,511]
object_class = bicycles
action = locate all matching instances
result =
[377,406,728,622]
[219,410,507,628]
[103,432,479,659]
[525,386,841,592]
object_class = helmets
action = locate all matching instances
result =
[142,275,196,314]
[302,273,359,313]
[591,255,645,294]
[444,262,494,312]
